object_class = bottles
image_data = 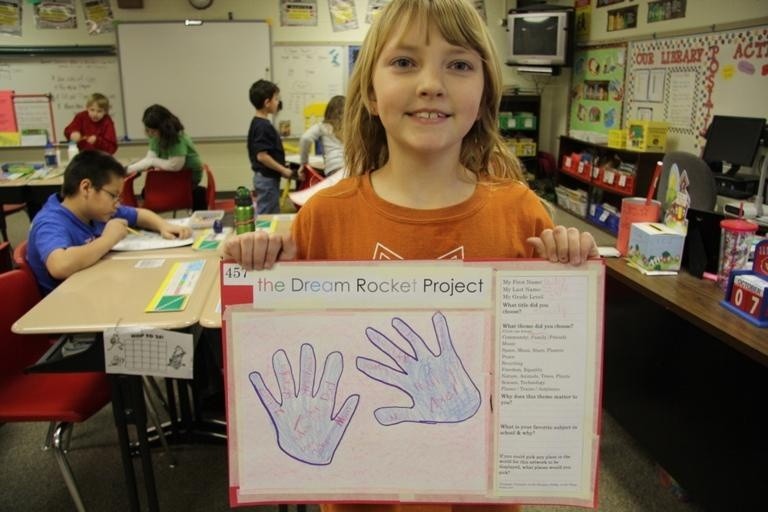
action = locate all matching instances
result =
[45,143,57,167]
[235,186,256,234]
[67,141,79,160]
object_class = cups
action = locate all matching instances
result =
[717,219,758,290]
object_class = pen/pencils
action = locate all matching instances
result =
[123,221,146,240]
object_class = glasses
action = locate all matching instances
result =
[92,181,125,203]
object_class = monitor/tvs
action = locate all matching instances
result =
[703,115,766,177]
[505,10,568,74]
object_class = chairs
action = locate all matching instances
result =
[118,169,142,209]
[11,234,35,277]
[0,266,114,512]
[143,169,193,220]
[200,162,237,210]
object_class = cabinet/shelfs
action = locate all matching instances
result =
[499,94,541,190]
[555,135,667,237]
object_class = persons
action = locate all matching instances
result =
[247,79,293,214]
[66,92,116,155]
[124,103,210,214]
[26,149,191,372]
[219,1,602,512]
[296,95,347,182]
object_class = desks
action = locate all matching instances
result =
[11,214,298,511]
[596,250,768,512]
[0,160,72,255]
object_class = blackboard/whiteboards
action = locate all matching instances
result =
[113,20,275,141]
[0,45,126,150]
[272,41,349,139]
